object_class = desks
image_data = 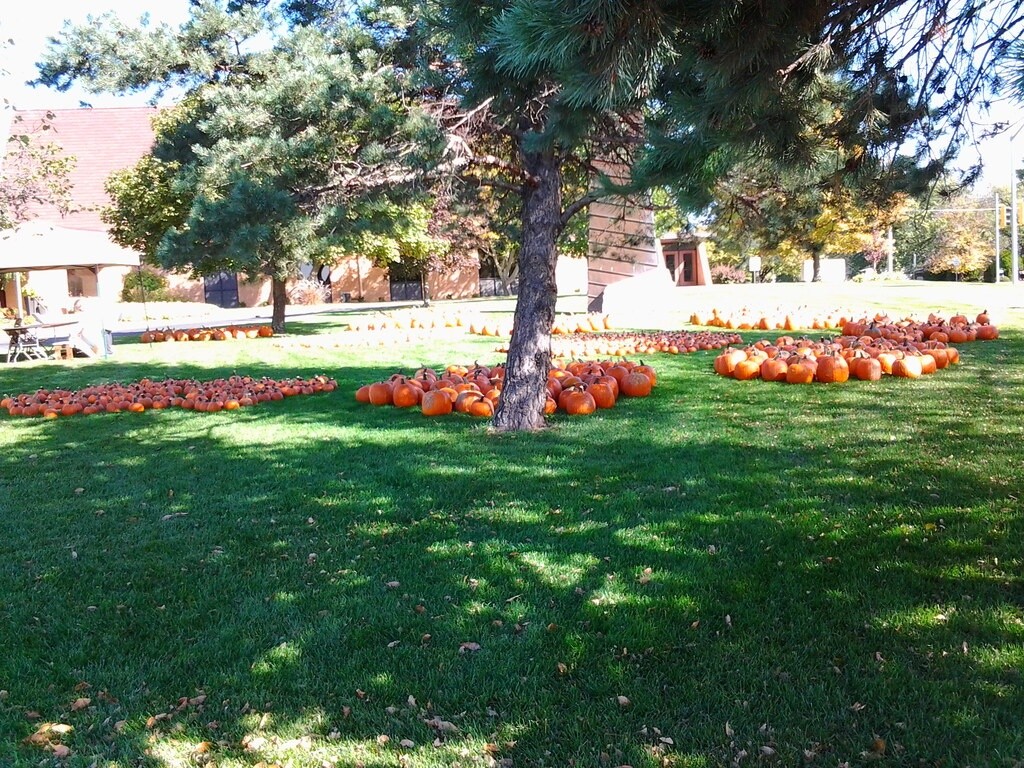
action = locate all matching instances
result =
[3,321,78,362]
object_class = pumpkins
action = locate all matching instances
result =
[344,305,999,418]
[0,324,338,418]
